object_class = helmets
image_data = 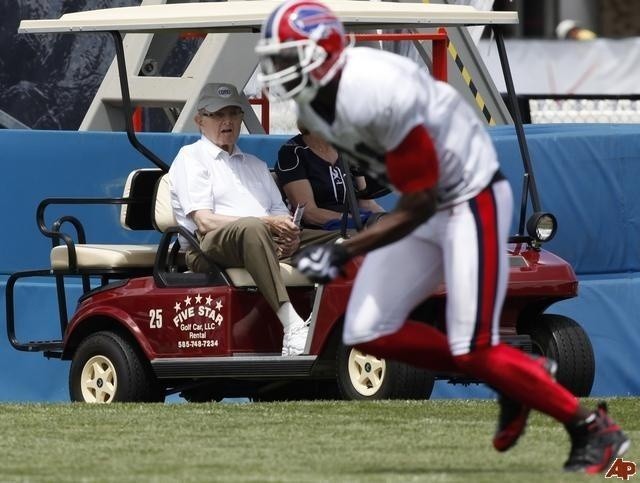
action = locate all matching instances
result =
[253,0,345,101]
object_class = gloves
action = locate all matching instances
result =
[296,243,351,284]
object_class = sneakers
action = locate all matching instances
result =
[282,324,309,358]
[564,407,631,474]
[493,351,557,450]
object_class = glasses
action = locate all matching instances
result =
[203,110,244,120]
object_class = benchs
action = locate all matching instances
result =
[50,168,317,295]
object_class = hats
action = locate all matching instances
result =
[197,84,242,113]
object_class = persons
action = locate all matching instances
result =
[553,16,595,39]
[278,112,392,235]
[168,82,310,355]
[254,0,632,475]
